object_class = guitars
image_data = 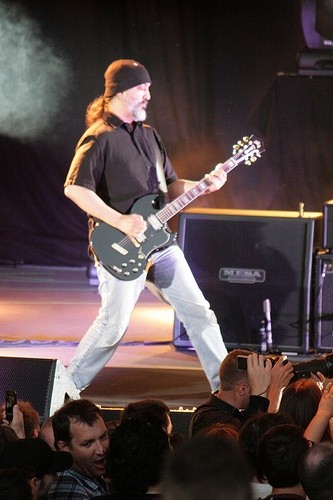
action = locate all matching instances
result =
[89,134,266,281]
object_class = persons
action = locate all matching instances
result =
[244,354,272,423]
[189,349,327,438]
[64,60,230,396]
[0,379,332,500]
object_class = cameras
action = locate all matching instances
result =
[265,353,333,385]
[236,355,248,371]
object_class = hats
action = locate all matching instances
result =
[14,438,74,473]
[103,60,152,97]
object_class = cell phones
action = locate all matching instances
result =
[5,391,18,421]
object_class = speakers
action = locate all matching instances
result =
[0,356,81,430]
[172,207,323,351]
